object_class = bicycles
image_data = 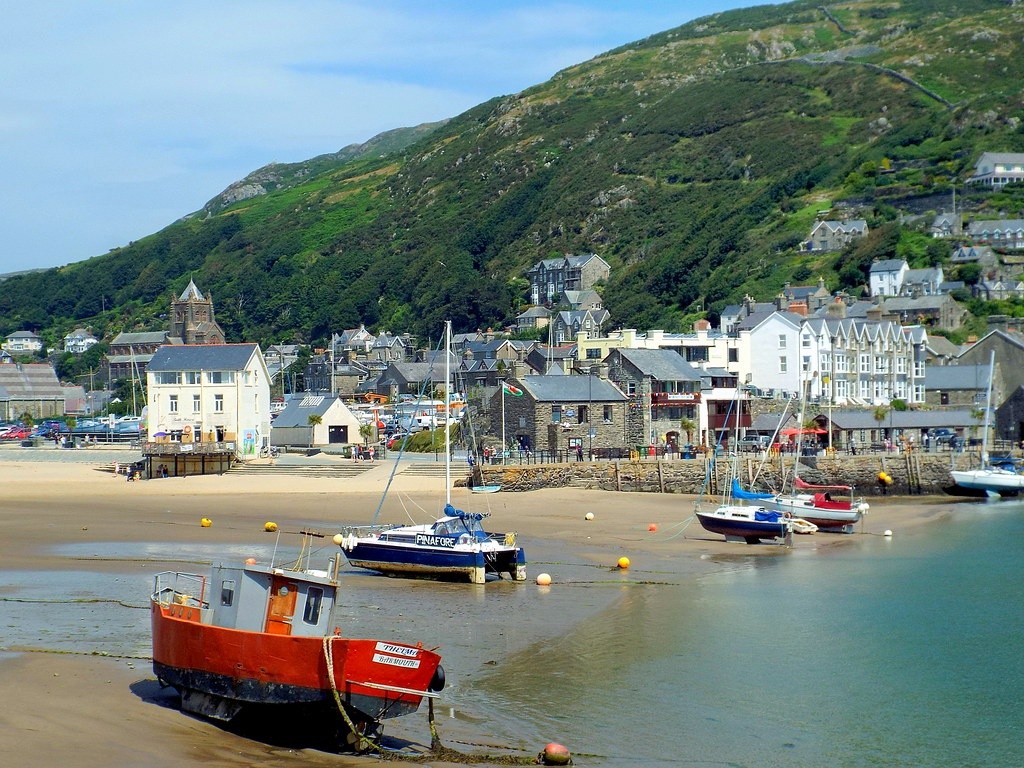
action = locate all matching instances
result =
[259,445,281,459]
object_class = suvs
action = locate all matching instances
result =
[733,435,771,453]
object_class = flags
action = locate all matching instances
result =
[503,381,523,397]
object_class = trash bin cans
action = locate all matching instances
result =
[636,445,648,457]
[648,445,654,456]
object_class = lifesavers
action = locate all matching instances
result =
[184,426,191,433]
[782,513,790,522]
[460,534,473,549]
[431,665,445,691]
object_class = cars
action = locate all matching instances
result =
[0,415,142,440]
[381,423,413,449]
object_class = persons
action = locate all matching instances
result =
[114,462,119,477]
[484,444,496,463]
[810,439,814,447]
[784,512,793,549]
[887,435,892,445]
[576,444,584,461]
[93,436,97,447]
[525,446,530,457]
[139,420,146,432]
[47,428,66,448]
[85,434,89,444]
[851,439,856,455]
[158,464,168,478]
[351,444,364,463]
[208,430,215,443]
[369,446,375,463]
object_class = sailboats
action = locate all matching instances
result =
[950,349,1024,492]
[693,362,869,541]
[337,319,527,583]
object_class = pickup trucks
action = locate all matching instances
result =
[927,429,955,446]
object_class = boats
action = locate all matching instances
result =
[467,476,503,494]
[150,529,446,745]
